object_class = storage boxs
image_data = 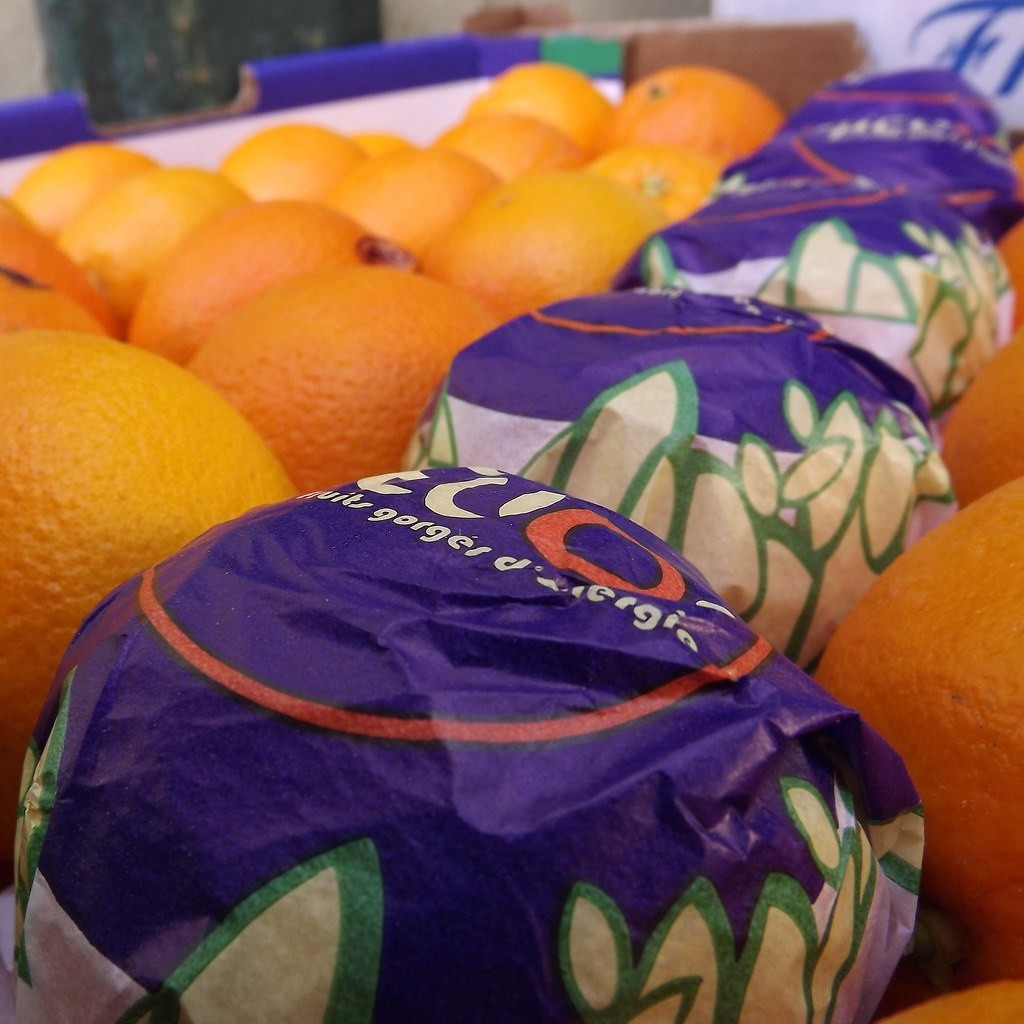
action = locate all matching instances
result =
[0,17,870,195]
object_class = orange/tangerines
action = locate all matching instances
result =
[0,61,1023,1023]
